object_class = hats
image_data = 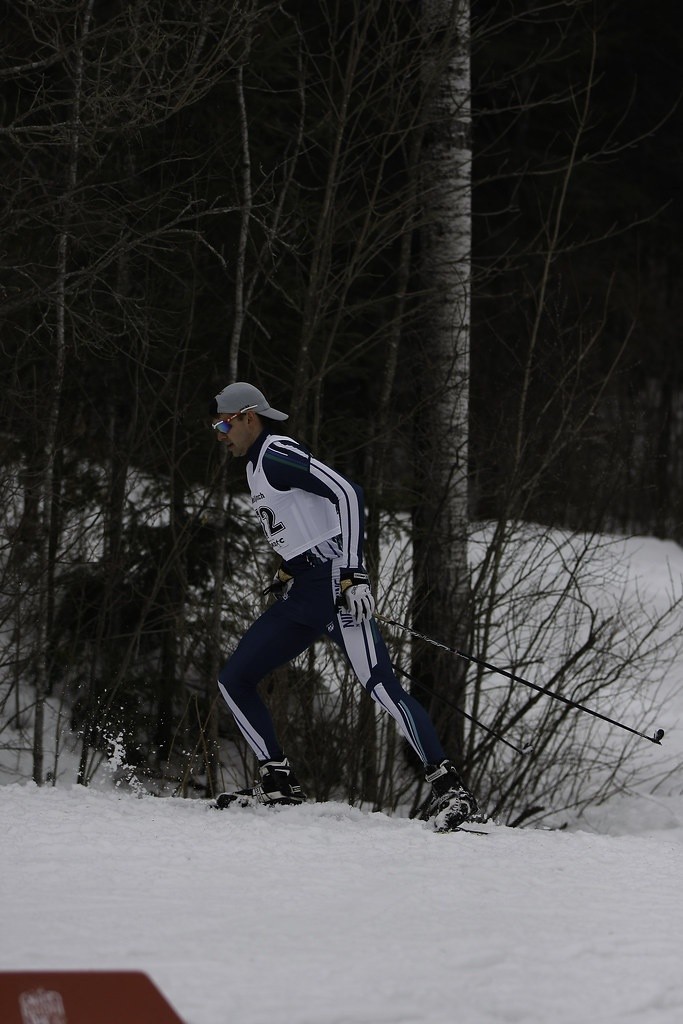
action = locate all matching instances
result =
[215,382,289,421]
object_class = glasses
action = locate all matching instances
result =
[212,404,258,434]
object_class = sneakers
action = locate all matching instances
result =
[425,760,478,832]
[216,756,306,810]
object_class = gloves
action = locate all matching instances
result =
[271,570,293,601]
[345,584,375,625]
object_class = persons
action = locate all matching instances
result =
[207,381,483,831]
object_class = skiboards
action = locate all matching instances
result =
[212,790,492,836]
